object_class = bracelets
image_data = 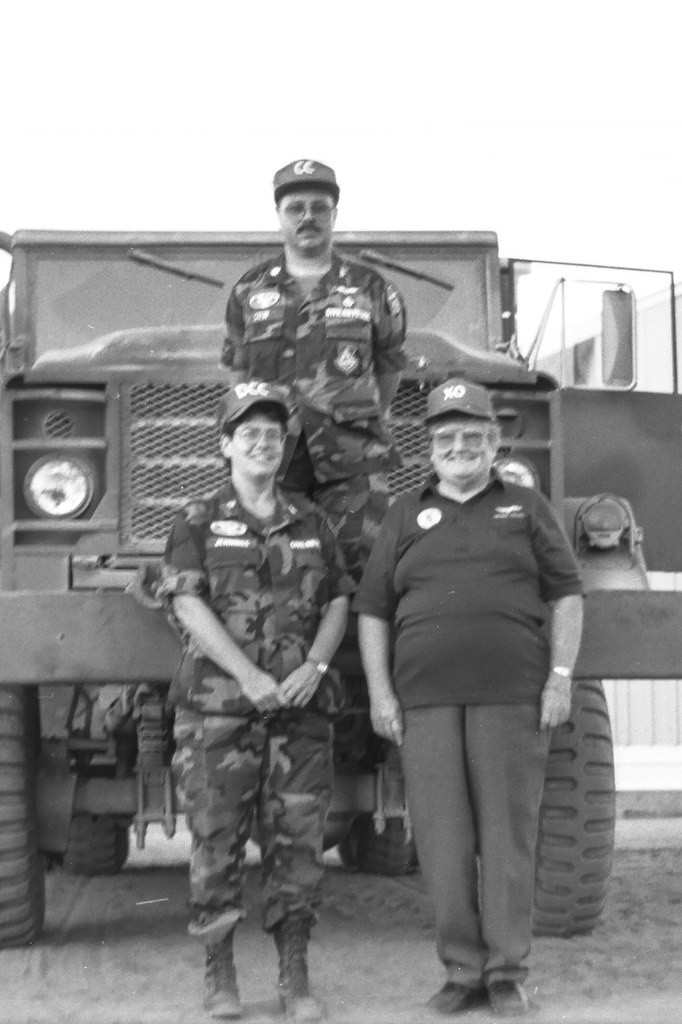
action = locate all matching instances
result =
[548,666,573,678]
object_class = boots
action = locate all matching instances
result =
[203,926,243,1016]
[274,916,322,1022]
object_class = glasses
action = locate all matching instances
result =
[229,426,287,446]
[279,203,336,221]
[431,428,490,449]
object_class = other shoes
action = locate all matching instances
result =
[486,979,529,1016]
[427,981,487,1012]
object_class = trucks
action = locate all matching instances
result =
[1,228,682,950]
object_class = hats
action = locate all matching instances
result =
[423,377,496,425]
[216,381,289,433]
[273,160,340,203]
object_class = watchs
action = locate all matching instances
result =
[304,656,329,676]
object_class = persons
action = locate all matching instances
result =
[219,160,408,585]
[162,382,357,1021]
[351,378,584,1021]
